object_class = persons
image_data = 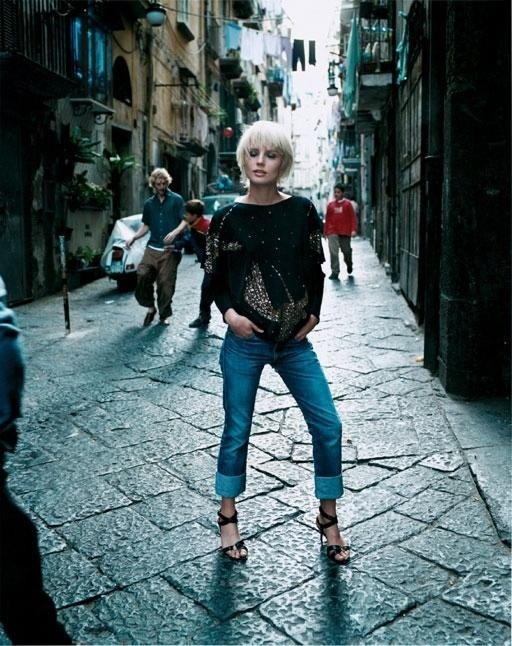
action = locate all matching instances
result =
[182,198,230,327]
[0,273,79,646]
[205,120,350,564]
[123,167,188,327]
[348,195,358,237]
[322,182,358,279]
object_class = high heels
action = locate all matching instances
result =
[218,508,248,562]
[316,505,350,564]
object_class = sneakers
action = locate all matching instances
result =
[189,319,209,328]
[329,273,338,279]
[347,261,352,273]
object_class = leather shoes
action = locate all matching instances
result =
[160,317,170,326]
[144,307,156,326]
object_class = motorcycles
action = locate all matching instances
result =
[102,212,151,291]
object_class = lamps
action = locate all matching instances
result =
[327,61,338,96]
[146,0,167,26]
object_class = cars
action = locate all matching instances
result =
[184,189,239,255]
